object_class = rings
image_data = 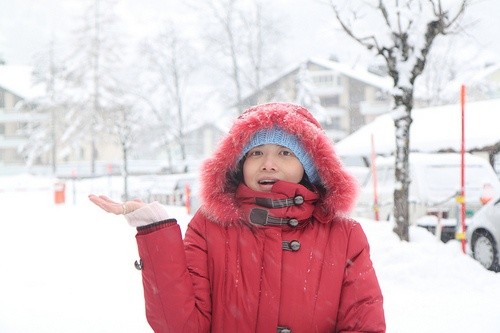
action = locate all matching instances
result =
[121,203,128,215]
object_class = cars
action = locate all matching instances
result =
[467,197,500,271]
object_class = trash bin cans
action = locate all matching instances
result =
[441,226,455,243]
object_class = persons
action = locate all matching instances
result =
[88,104,386,333]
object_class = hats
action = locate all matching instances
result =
[236,124,320,185]
